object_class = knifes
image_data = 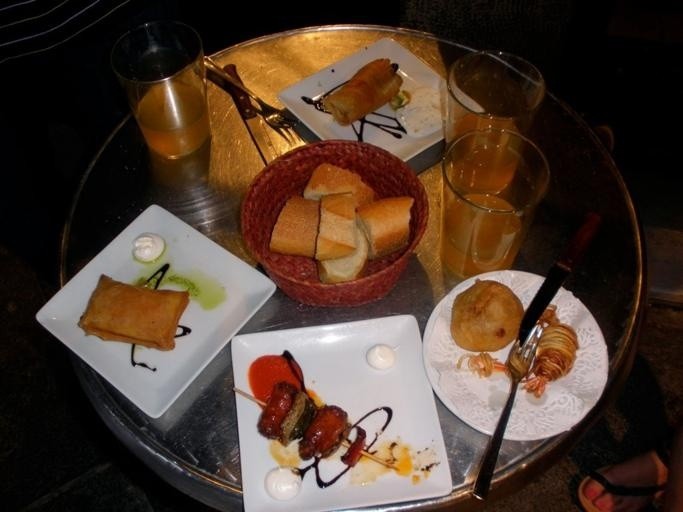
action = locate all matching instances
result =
[221,62,275,164]
[519,213,602,347]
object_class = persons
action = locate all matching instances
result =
[577,431,683,511]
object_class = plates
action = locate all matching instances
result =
[35,203,277,418]
[231,314,453,512]
[275,38,486,162]
[423,269,609,442]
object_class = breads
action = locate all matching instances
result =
[320,58,404,125]
[76,274,190,352]
[450,277,525,353]
[269,162,416,283]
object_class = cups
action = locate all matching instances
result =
[445,50,545,195]
[110,21,211,157]
[442,127,551,279]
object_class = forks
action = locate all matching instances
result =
[205,55,299,129]
[471,322,544,500]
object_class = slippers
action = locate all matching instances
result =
[577,465,670,512]
